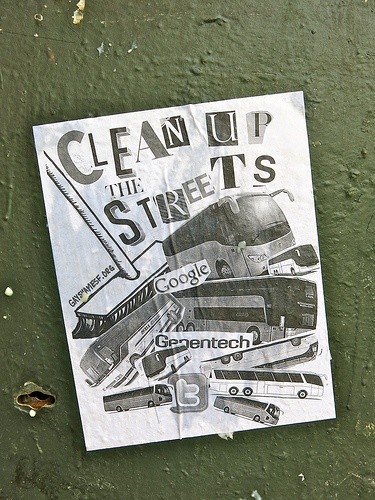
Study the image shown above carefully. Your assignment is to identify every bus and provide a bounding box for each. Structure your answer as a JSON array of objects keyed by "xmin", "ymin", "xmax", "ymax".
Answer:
[{"xmin": 77, "ymin": 187, "xmax": 330, "ymax": 426}]
